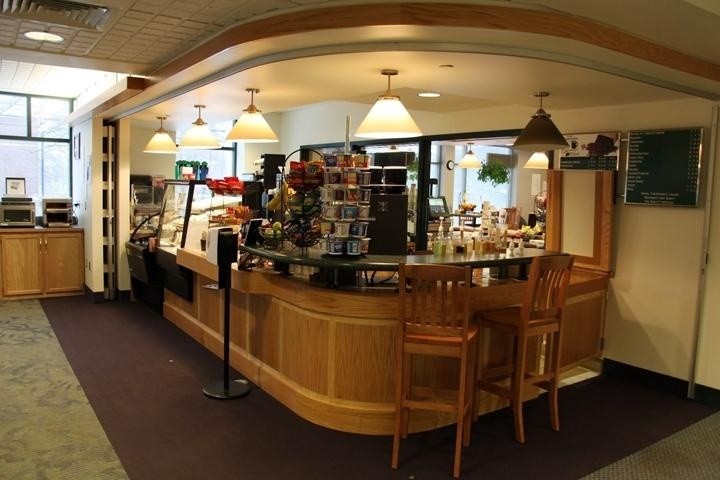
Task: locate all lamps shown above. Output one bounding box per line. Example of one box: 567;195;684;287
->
142;117;178;153
458;142;482;168
524;151;549;170
225;88;280;143
354;69;423;140
512;91;570;151
179;105;223;149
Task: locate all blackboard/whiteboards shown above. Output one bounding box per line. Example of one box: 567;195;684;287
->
624;126;703;207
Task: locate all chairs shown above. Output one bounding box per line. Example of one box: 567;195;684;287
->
473;253;575;444
501;207;522;228
391;262;480;479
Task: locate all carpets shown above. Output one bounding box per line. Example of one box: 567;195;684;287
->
0;298;130;480
581;411;719;479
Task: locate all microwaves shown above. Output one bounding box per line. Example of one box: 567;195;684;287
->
0;201;36;229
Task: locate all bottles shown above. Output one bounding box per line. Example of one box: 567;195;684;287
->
433;216;523;257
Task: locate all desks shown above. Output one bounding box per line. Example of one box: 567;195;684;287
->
450;213;482;239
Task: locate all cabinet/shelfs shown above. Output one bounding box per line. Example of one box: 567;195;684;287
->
0;226;84;301
